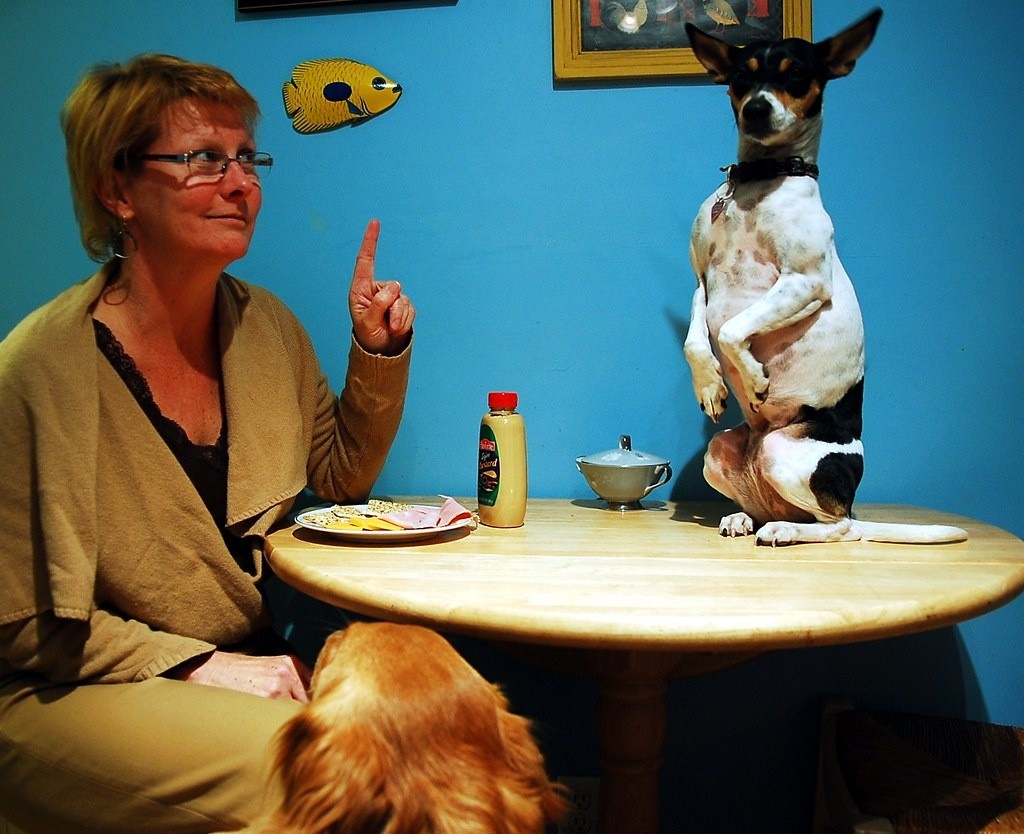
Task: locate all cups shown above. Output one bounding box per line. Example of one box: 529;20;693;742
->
577;435;673;512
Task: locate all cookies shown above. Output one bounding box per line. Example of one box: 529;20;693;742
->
302;500;413;526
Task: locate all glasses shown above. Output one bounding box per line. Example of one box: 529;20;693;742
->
130;149;274;180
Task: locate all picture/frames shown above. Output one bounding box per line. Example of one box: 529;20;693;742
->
551;0;812;81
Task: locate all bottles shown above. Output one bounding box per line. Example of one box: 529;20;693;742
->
479;392;527;528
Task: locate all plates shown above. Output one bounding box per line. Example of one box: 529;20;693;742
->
294;505;473;543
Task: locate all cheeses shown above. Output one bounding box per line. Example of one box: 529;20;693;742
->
325;516;404;531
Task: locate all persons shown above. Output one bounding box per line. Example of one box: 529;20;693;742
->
0;53;415;834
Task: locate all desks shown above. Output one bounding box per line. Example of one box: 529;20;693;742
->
264;501;1024;834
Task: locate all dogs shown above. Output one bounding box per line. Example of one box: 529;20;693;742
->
682;7;969;548
202;618;570;834
